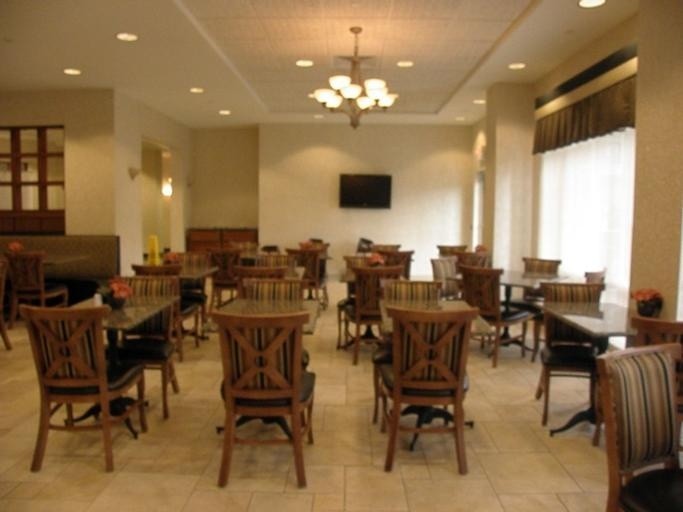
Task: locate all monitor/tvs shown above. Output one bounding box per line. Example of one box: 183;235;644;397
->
339;174;391;209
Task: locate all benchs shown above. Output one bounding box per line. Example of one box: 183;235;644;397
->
1;236;120;306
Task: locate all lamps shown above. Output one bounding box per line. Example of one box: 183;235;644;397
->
313;27;400;129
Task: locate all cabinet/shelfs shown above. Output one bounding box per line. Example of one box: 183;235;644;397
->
190;229;257;253
